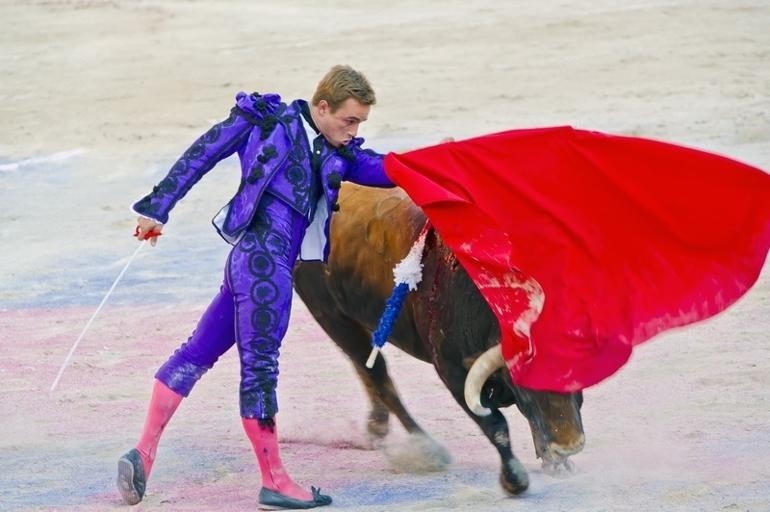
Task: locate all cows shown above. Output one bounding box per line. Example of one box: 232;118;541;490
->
292;182;586;494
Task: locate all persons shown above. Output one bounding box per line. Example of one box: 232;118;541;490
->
117;64;455;510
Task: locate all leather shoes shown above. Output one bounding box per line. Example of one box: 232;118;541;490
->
117;449;146;504
257;485;332;510
